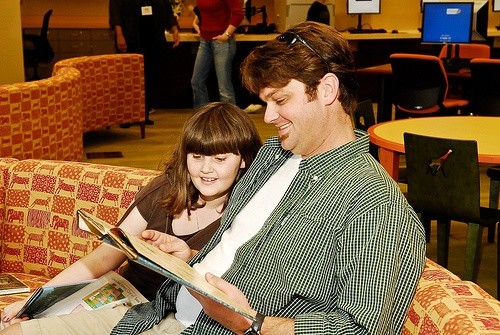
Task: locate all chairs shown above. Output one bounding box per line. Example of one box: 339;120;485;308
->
351;41;500;280
23;10;53;79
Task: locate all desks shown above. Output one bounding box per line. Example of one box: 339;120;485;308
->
355;62;471;124
366;116;500;184
167;29;420;42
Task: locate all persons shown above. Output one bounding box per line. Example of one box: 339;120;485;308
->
0;102;263;332
1;22;427;335
191;0;245;110
109;0;179;129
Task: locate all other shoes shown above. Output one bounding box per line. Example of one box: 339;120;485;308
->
144;119;154;125
242;104;262;113
119;122;132;128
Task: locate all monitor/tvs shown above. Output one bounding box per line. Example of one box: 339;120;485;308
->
347;0;381;29
422;2;475;44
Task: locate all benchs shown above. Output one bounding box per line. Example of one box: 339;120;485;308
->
0;53;146;160
0;158;500;335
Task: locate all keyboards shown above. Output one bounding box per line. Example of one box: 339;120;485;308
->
349;29;387;33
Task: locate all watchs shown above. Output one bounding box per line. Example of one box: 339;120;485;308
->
244;313;266;335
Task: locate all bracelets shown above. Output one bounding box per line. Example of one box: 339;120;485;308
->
225;32;232;38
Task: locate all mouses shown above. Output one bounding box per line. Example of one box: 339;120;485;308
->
392;30;398;33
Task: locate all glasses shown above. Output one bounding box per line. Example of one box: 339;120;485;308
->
275;31;330;74
